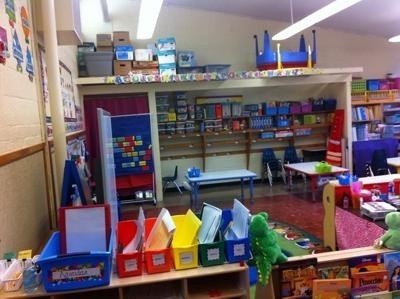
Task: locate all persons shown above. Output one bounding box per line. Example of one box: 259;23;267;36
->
390;265;400;291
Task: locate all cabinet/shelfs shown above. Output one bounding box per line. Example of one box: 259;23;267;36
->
159;110;334;175
351;98;400;141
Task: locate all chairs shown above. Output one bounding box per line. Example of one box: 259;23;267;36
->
162;165;183;196
365;149;391;176
262;147;307;186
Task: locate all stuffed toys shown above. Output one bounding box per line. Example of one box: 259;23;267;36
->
373;211;400;251
248;211;288;288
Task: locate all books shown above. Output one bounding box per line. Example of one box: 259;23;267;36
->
362;200;398;212
352;105;383;142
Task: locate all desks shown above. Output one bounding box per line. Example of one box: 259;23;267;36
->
283;162;350;201
184;168;257;212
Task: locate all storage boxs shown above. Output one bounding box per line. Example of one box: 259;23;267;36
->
77;31;232;77
